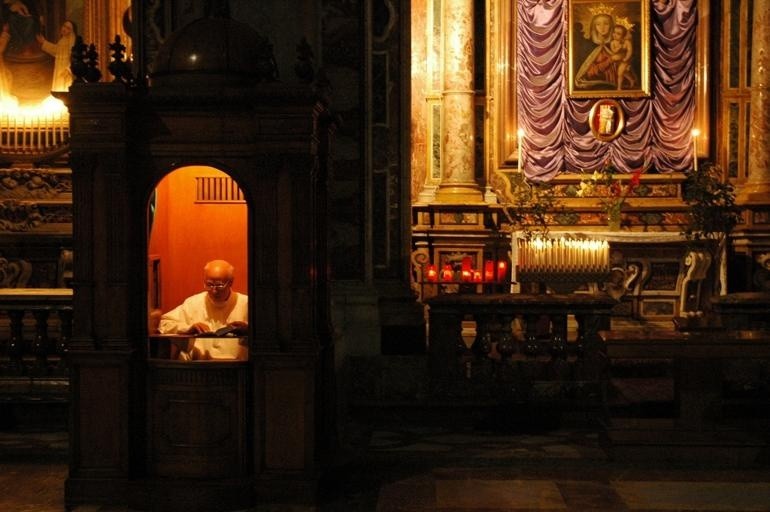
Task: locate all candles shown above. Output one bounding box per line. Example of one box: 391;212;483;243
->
516;236;609;273
425;256;508;284
1;116;63;155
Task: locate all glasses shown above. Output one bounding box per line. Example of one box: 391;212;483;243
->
203;281;231;290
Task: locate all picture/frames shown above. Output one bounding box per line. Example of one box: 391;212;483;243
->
567;0;654;100
0;0;108;134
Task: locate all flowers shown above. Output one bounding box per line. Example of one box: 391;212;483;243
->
575;166;644;222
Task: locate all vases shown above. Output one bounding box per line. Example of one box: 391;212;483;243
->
606;216;620;232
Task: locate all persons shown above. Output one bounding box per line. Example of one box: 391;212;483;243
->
574;14;636;91
157;260;248;361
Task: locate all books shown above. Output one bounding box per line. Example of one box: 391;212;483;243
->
194;325;237;337
